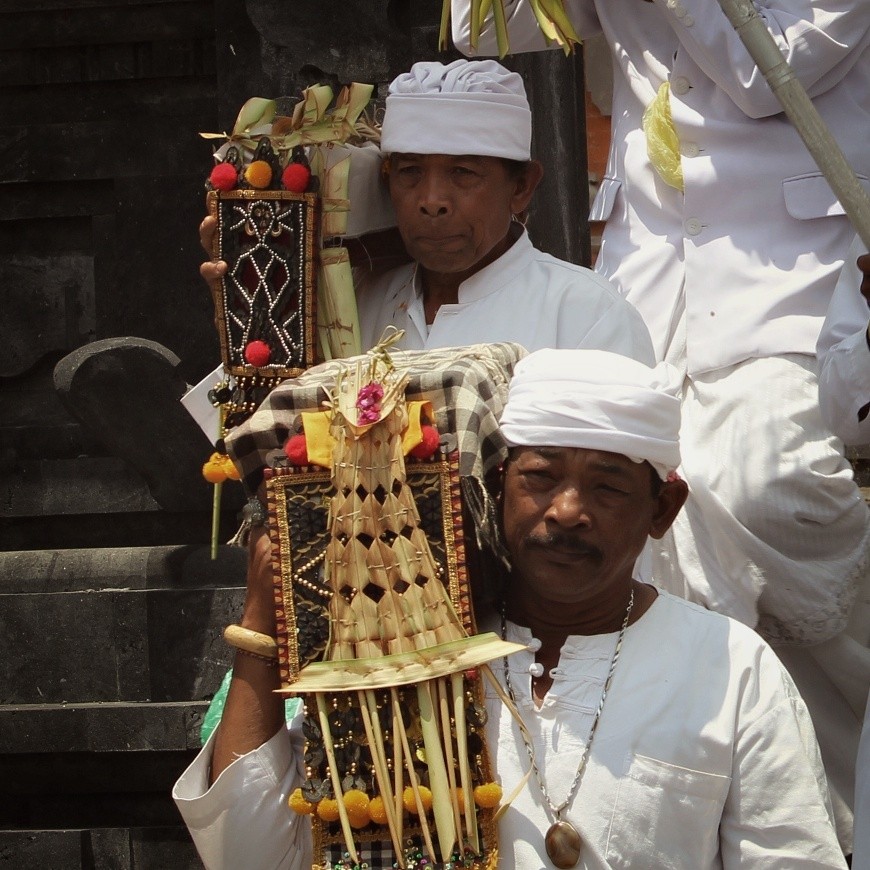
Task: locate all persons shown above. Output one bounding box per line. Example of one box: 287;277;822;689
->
448;2;870;870
172;356;850;869
200;60;657;370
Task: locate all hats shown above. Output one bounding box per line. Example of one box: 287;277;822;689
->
381;61;534;162
501;350;682;481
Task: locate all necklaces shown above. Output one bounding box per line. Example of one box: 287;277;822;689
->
493;579;640;869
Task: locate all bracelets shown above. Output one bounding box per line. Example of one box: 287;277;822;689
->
223;624;279;661
